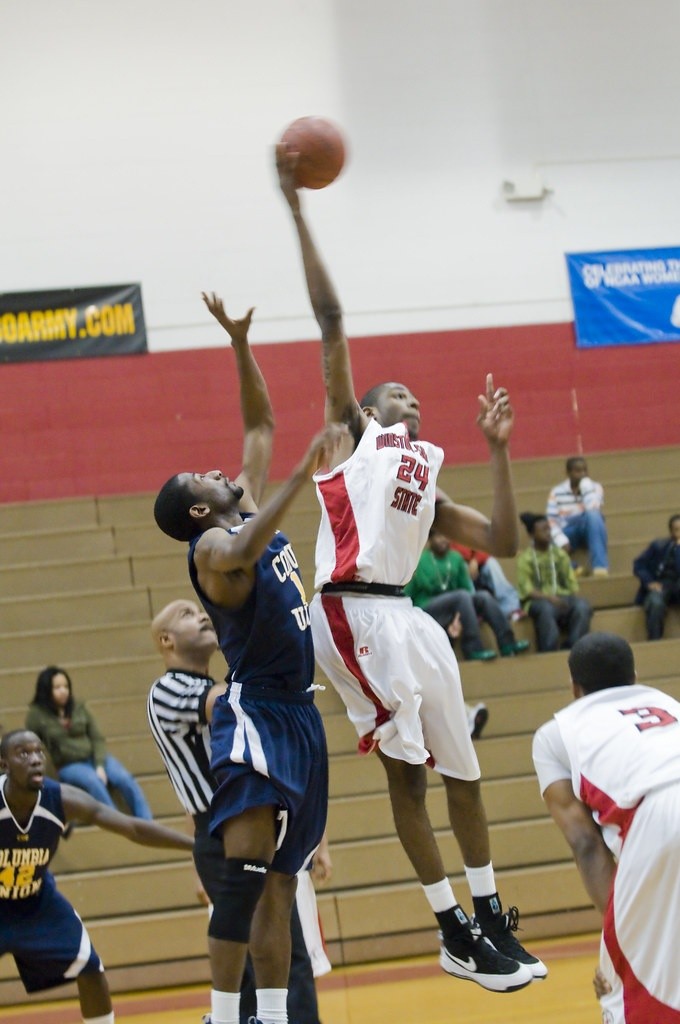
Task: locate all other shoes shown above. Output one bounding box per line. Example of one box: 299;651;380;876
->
470;702;489;742
469;650;497;661
574;566;585;578
501;640;530;658
593;566;608;578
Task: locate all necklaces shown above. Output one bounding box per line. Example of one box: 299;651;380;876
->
431;549;451;589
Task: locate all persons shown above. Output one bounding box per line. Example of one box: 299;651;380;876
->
531;630;680;1024
632;514;680;642
0;729;194;1024
153;291;341;1024
450;543;521;623
405;530;530;661
148;599;320;1024
24;666;153;821
275;143;549;992
516;510;593;653
547;456;610;576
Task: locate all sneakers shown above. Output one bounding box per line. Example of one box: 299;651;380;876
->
471;906;549;978
437;923;533;993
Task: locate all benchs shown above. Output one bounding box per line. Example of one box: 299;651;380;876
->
0;448;680;1006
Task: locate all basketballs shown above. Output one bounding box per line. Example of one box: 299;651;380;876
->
276;115;351;192
